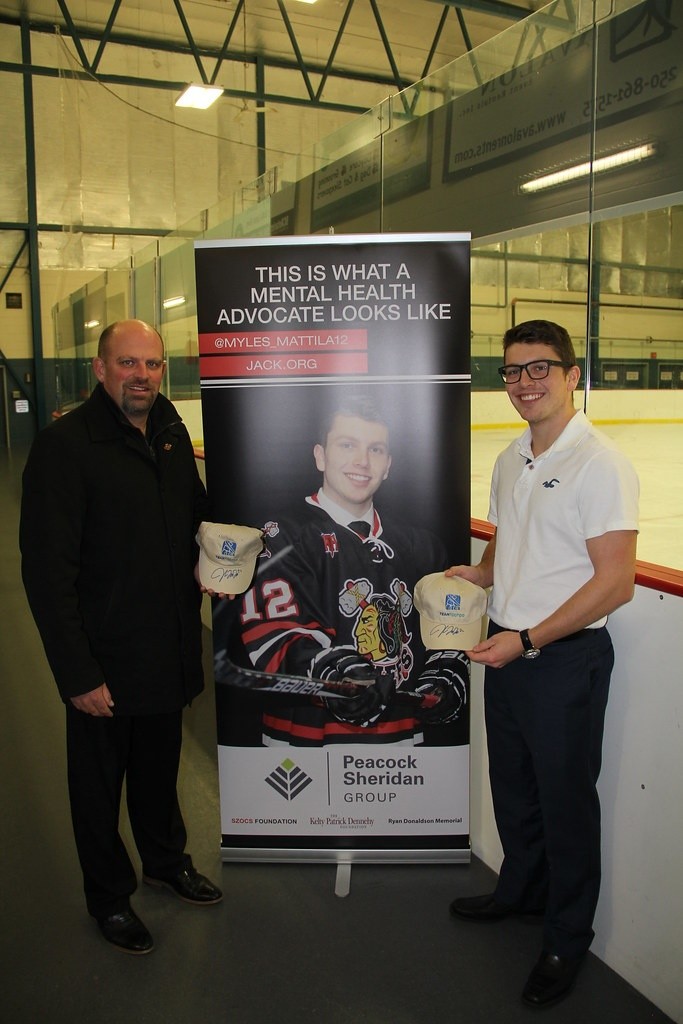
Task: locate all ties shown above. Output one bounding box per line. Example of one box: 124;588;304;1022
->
347;521;372;539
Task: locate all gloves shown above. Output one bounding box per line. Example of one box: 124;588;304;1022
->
414;648;471;725
305;643;397;728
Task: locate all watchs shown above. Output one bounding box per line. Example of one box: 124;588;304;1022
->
520;629;541;661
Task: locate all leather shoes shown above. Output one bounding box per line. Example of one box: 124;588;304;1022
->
521;942;591;1008
448;892;545;923
90;895;153;954
142;866;223;904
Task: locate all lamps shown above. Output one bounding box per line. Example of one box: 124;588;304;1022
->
176;83;225;110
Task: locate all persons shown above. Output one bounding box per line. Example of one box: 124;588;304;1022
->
20;319;237;954
446;321;640;1009
232;395;470;747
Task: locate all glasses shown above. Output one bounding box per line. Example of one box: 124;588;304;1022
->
497;359;573;383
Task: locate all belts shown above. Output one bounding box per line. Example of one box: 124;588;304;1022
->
490;618;606;642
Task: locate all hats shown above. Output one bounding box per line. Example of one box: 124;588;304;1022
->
195;522;263;594
413;572;488;652
